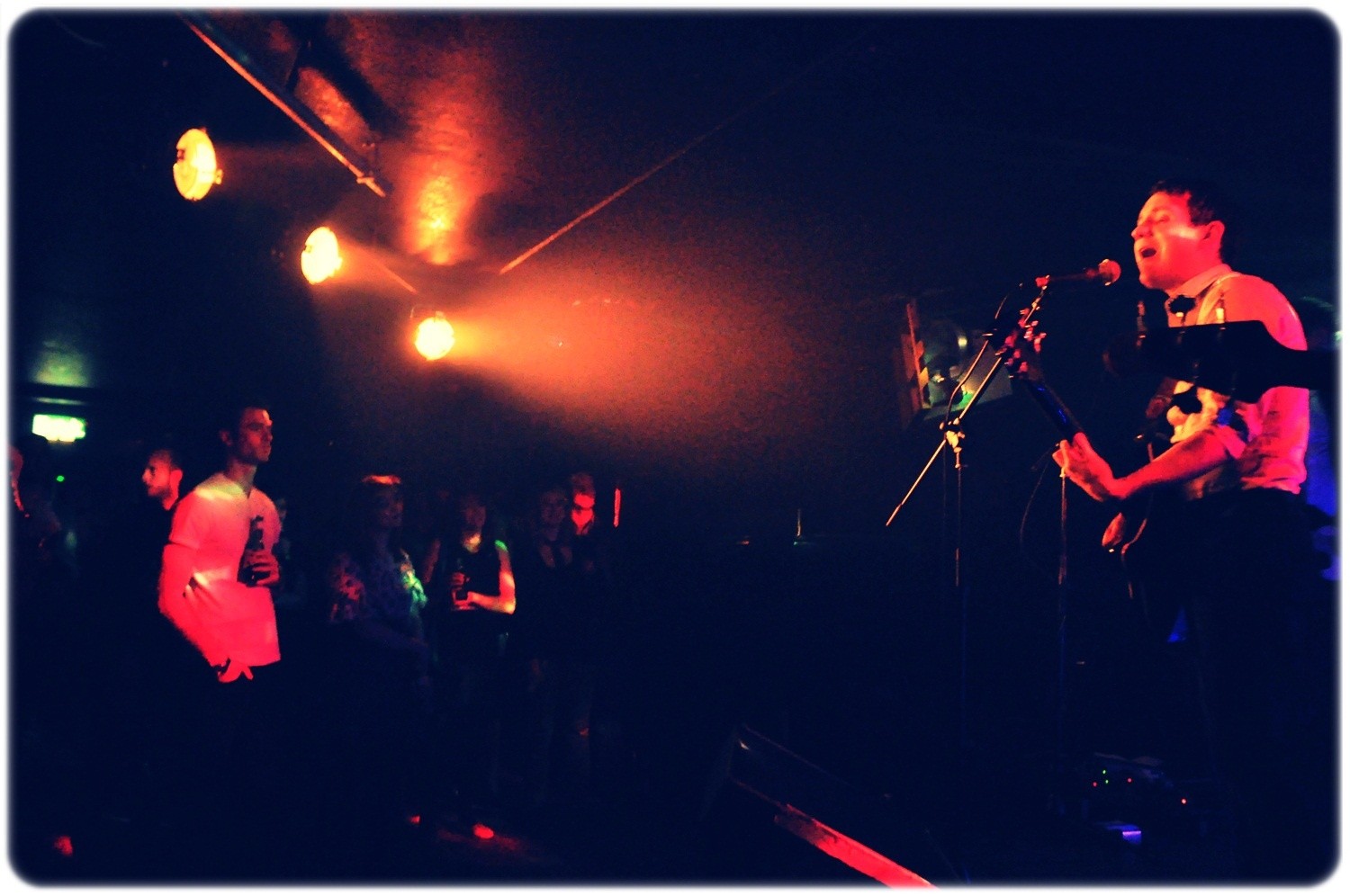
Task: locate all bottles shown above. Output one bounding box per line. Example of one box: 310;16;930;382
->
239;516;264;586
455;558;468;599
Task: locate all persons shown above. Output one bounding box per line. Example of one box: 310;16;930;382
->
10;396;623;852
1050;172;1311;885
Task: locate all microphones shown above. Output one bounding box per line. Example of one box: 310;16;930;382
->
1035;258;1122;287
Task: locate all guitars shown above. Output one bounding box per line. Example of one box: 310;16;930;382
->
989;295;1184;596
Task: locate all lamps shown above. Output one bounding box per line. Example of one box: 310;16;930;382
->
124;109;223;210
400;301;454;363
274;214;345;284
